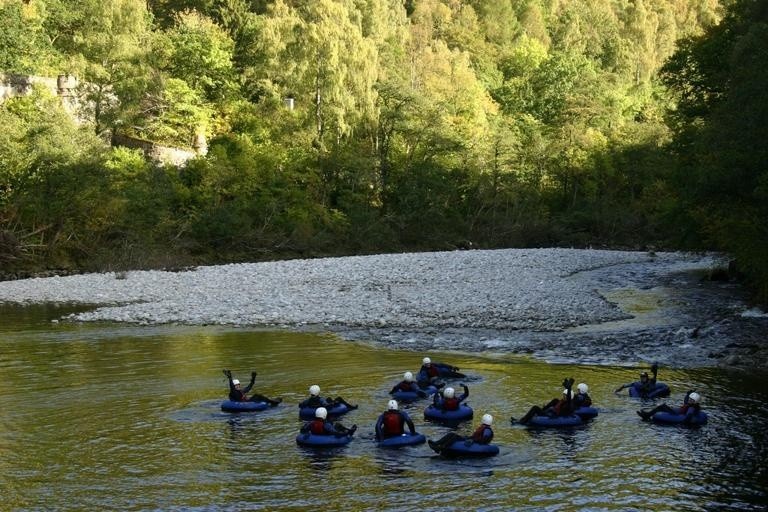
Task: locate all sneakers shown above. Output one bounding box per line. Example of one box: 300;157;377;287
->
636;410;650;421
349;424;357;435
272;397;282;407
510;416;520;425
427;439;441;454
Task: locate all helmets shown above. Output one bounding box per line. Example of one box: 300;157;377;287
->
388;400;399;410
423;357;431;364
315;407;327;420
233;379;240;386
481;413;493;426
444;387;455;399
404;372;413;382
577;382;589;394
564;388;576;400
309;385;321;397
639;372;648;378
689;392;700;401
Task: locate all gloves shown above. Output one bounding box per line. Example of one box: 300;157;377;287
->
454;366;460;371
251;372;256;378
418;390;427;399
683;415;692;424
687;388;697;394
464;439;474;446
222;369;232;376
459;383;466;387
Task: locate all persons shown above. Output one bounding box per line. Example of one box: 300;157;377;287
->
616;365;658;396
376;399;415;438
223;368;282;407
428;413;494;454
388;371;427;400
510;378;575;426
299;385;358;411
303;406;357;437
574;383;592;408
433;383;469;411
636;390;701;422
419;357;454;388
